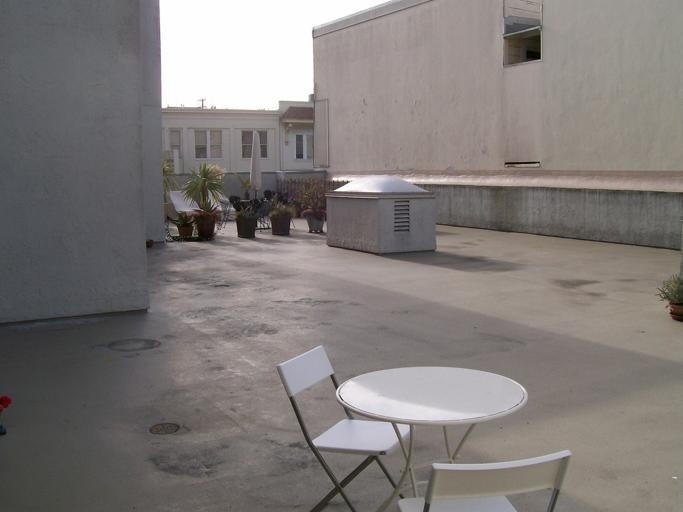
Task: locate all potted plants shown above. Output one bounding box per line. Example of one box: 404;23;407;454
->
164;166;327;237
654;272;683;322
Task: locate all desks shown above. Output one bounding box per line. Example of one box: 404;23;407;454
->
335;365;527;512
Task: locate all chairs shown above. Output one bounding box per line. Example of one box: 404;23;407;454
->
275;344;413;512
396;449;573;512
